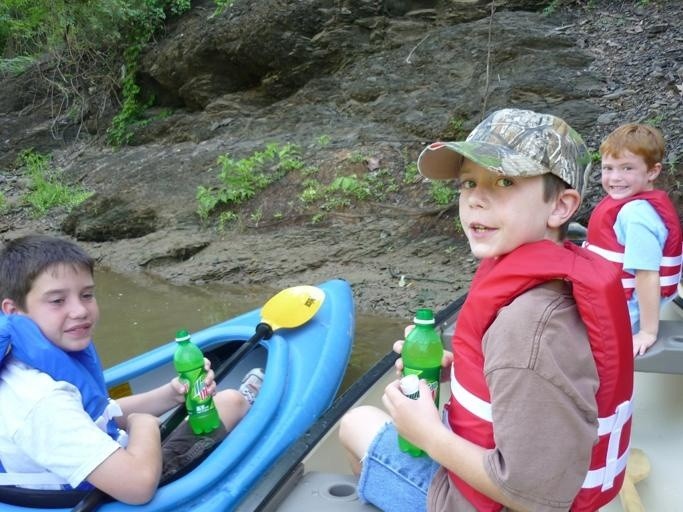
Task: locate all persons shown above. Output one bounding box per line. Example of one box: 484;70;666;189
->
337;105;637;512
578;122;683;358
0;231;267;512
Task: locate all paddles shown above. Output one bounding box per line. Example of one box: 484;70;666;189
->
74;285;325;508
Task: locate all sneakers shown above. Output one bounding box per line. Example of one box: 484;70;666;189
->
240;366;264;402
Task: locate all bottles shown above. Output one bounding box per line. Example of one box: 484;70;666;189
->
172;329;221;435
399;308;447;458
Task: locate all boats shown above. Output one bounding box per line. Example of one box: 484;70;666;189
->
231;218;682;512
1;276;356;512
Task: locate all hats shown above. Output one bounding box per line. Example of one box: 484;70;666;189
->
418;107;593;203
